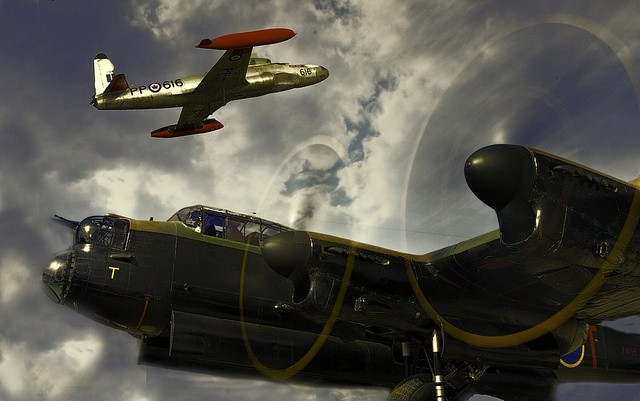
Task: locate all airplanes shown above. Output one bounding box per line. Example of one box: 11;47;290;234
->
42;144;640;401
91;27;329;138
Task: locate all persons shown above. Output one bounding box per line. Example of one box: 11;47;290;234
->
199;215;216;237
